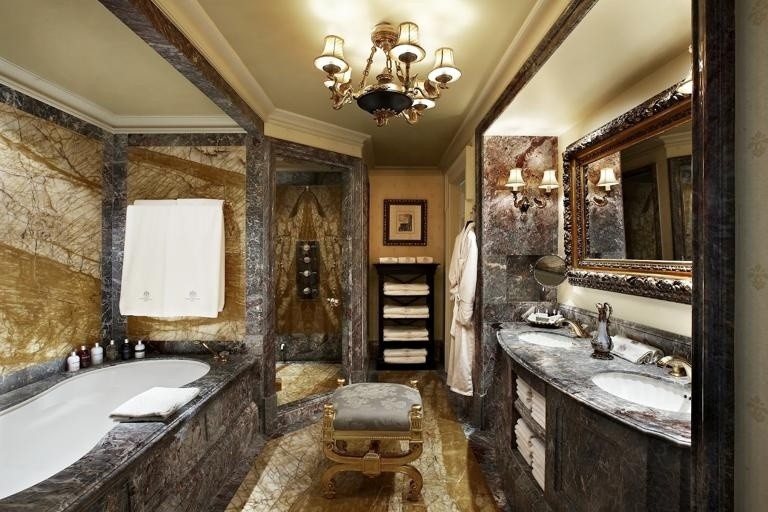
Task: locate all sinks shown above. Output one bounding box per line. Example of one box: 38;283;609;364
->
593;368;692;413
518;330;573;347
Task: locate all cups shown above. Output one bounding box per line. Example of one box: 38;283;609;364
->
219;351;229;362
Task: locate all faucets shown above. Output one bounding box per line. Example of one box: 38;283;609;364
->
193;339;220;359
556;315;590;338
657;354;692;380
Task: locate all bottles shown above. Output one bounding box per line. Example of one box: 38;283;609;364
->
590;300;615;359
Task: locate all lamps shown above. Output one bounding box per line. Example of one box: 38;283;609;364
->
587;166;620;207
504;167;559;213
313;21;464;130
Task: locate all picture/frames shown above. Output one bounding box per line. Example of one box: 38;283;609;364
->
382;198;429;246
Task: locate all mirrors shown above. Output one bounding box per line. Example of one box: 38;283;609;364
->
560;72;693;305
528;253;568;287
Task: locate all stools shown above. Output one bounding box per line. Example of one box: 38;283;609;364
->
319;375;426;500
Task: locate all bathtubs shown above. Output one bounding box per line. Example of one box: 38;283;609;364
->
0;356;211;503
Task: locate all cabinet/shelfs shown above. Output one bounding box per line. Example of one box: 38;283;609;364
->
371;261;441;370
508;370;545;494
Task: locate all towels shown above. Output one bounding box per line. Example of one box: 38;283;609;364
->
382;281;429;365
108;386;201;421
513;376;546;491
380;256;434;264
116;197;226;320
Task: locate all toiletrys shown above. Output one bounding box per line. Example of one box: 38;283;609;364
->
67;339;145;372
